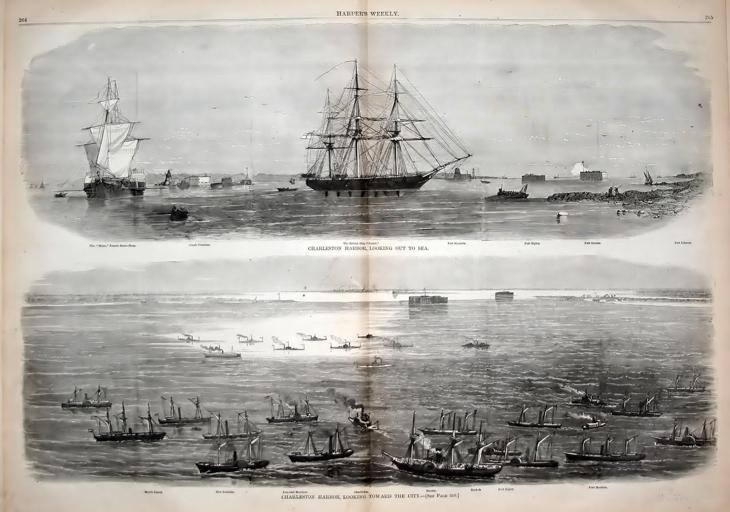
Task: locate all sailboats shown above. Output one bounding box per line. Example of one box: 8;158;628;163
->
566;372;716;462
61;384;210;441
74;73;146;198
382;405;563;478
303;56;471;196
287;422;354;462
195;407;270;477
265;393;318;424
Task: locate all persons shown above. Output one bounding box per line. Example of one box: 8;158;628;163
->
614;187;619;197
608;186;613;197
349;412;367;428
424;448;448;463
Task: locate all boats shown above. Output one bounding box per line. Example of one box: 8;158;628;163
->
486;184;529;201
463;338;490;349
178;332;414;368
346;402;379;432
277;187;297;192
170;204;187;221
